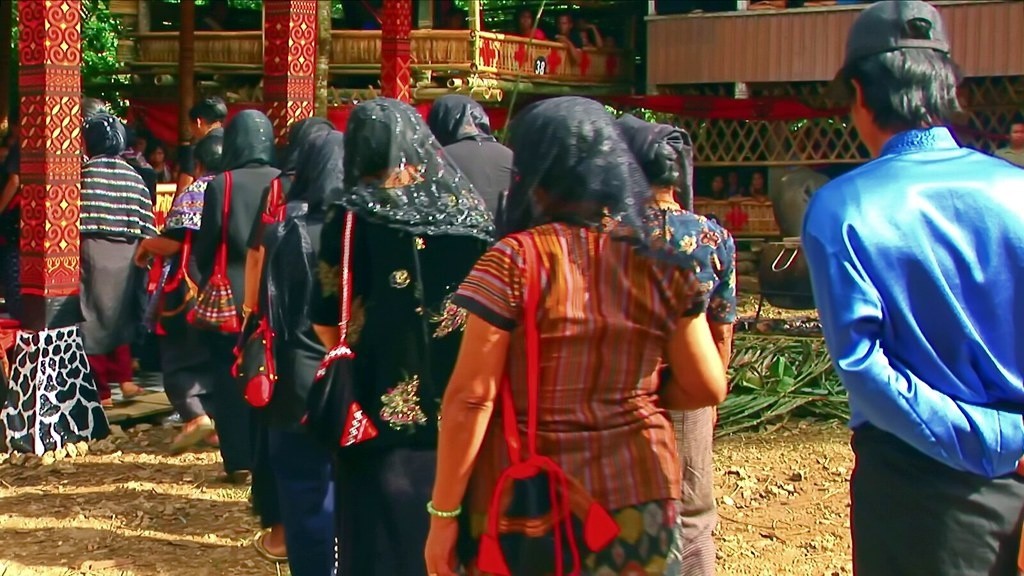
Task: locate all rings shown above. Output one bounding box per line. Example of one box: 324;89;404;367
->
429;573;437;576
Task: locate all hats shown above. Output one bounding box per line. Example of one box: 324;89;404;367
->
828;0;953;104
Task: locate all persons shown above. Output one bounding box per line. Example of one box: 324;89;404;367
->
710;169;768;203
511;8;615;63
993;121;1024;168
0;93;738;576
801;0;1024;576
747;0;788;11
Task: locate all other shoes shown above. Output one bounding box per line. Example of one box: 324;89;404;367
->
166;413;216;455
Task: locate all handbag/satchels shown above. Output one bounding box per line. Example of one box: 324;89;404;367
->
230;318;278;408
299;345;379;450
476;458;620;576
155;264;241;338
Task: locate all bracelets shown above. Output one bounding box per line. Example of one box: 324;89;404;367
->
426;501;462;518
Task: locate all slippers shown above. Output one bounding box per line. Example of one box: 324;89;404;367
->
100;403;114;410
252;527;291;563
124;383;144;400
